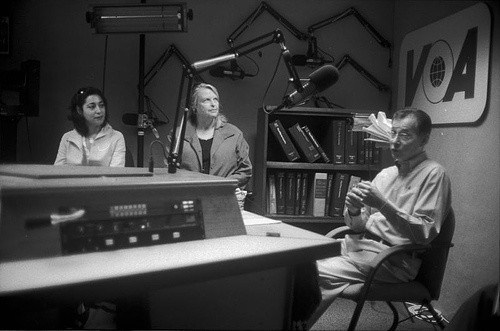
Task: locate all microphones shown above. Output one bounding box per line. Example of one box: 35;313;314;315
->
122;113;168;125
209;66;256;79
267;64;338;114
292;54;333;66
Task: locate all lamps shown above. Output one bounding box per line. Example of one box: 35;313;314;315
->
86;3;193;34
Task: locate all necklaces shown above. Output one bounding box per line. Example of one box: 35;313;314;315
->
197;130;210;144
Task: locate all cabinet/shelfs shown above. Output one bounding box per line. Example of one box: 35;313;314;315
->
252;105;384;235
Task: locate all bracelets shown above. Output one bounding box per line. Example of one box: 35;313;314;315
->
379;200;388;211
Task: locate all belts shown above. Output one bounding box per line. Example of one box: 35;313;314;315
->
364;230;393;248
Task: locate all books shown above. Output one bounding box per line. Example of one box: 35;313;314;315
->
267;117;381;217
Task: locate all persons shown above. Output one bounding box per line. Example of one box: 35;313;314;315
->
54;86;126;166
163;83;252;188
297;107;451;331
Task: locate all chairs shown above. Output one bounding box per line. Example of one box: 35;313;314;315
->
317;205;455;331
